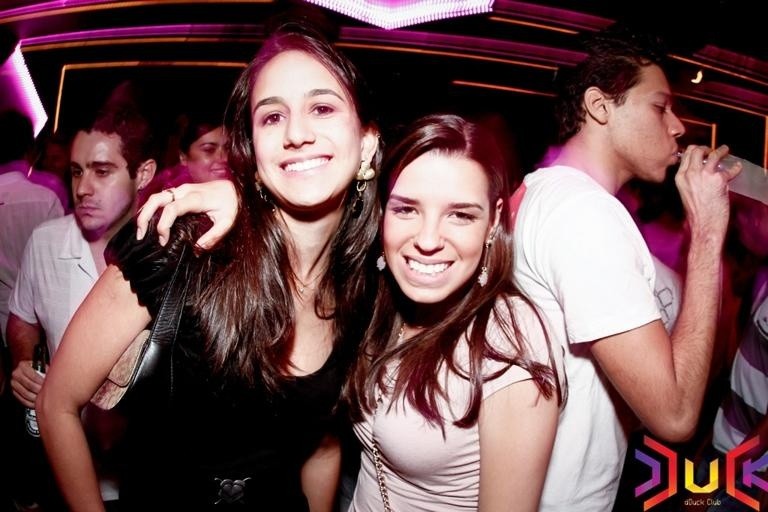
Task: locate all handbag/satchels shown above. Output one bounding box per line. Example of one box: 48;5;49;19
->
81;215;202;442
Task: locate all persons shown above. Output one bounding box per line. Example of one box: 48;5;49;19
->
1;24;768;512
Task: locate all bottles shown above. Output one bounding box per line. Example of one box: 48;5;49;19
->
677;148;768;205
24;343;50;439
